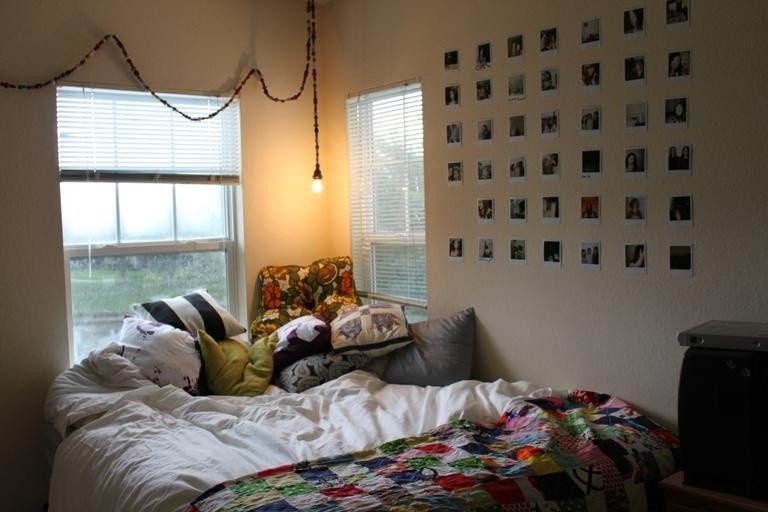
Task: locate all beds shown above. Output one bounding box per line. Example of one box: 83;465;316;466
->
46;378;680;511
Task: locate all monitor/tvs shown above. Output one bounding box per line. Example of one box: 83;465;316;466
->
677;348;768;502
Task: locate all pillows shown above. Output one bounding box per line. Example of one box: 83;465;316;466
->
196;328;278;395
371;350;391;378
329;305;415;359
305;255;354;306
117;315;203;395
132;288;247;341
383;307;475;385
271;313;329;373
251;303;315;342
313;292;364;323
259;263;312;306
275;350;370;395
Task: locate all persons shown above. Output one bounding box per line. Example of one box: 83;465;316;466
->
446;2;691;269
